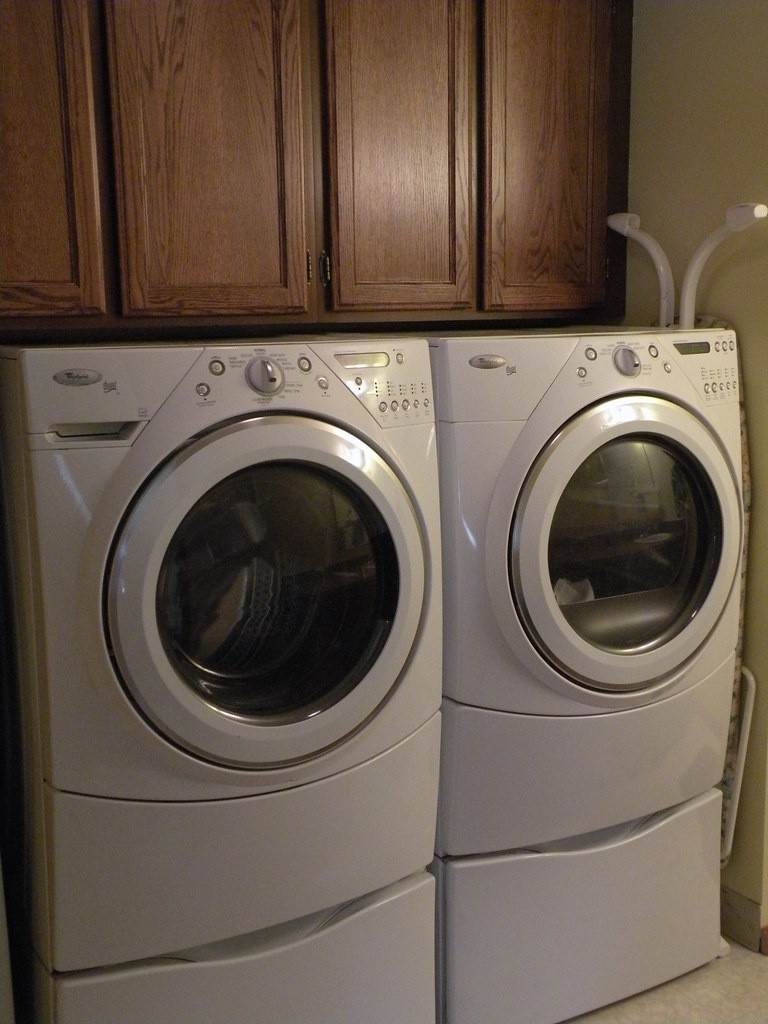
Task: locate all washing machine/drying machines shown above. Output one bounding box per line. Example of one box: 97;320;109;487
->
1;335;444;1024
421;319;751;1022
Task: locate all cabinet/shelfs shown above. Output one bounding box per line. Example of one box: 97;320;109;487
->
2;1;628;325
